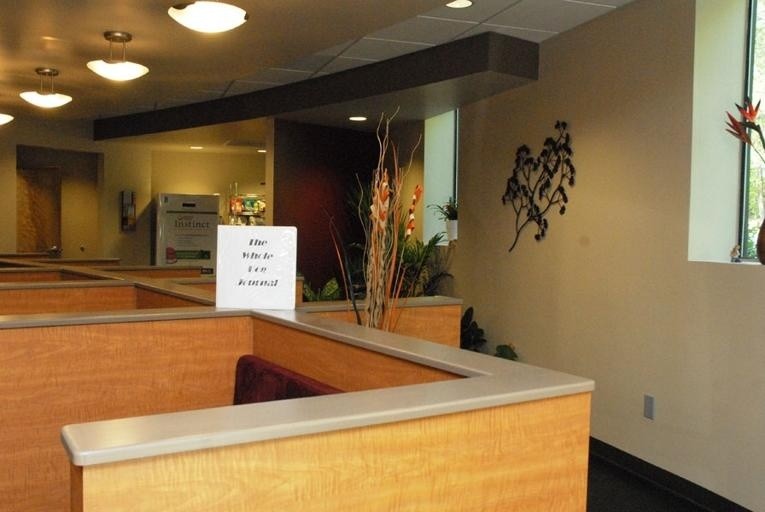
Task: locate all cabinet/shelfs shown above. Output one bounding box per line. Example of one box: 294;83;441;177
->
229;194;268;226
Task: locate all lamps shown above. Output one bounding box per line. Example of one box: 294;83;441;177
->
19;67;73;109
86;31;149;81
167;0;251;34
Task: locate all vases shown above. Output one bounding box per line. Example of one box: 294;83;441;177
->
756;218;764;264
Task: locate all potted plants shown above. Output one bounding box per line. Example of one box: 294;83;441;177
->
426;197;459;245
